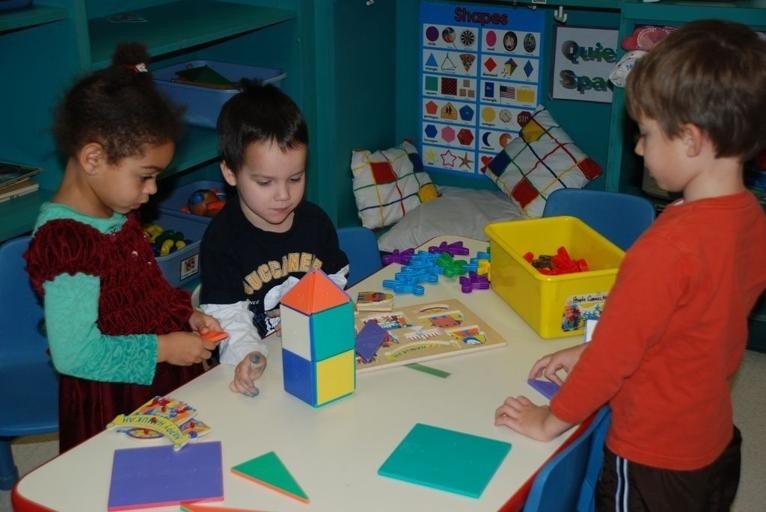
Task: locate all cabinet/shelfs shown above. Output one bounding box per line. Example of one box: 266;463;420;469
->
2;0;317;246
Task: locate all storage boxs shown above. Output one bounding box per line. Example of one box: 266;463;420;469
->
485;215;627;340
139;181;228;287
147;60;288;132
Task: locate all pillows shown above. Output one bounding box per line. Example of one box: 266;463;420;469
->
349;100;602;253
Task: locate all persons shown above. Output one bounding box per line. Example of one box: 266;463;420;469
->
196;81;352;398
495;19;766;511
20;63;223;457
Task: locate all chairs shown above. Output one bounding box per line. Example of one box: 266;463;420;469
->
0;237;63;493
522;407;612;512
543;188;656;253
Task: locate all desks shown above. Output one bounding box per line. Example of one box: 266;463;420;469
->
13;233;589;511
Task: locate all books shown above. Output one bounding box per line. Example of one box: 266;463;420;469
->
0;161;43;204
